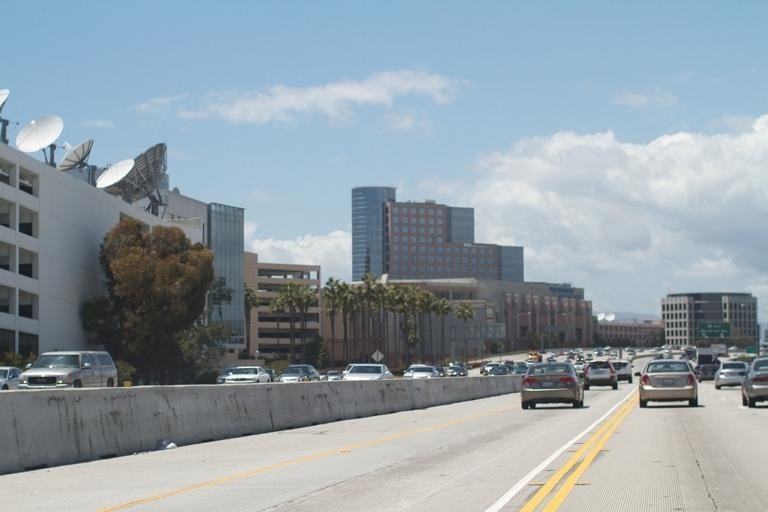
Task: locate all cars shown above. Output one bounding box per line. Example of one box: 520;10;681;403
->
698;364;720;382
573;364;585;377
527;345;695;362
634;359;698;407
0;365;25;390
610;360;634;383
741;357;768;408
520;361;585;408
692;366;700;377
714;360;749;389
731;352;756;362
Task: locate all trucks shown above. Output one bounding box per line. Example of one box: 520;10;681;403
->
696;346;719;365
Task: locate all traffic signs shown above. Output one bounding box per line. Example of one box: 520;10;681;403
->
696;321;730;338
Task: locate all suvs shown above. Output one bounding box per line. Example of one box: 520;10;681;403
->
582;359;619;391
16;350;119;391
